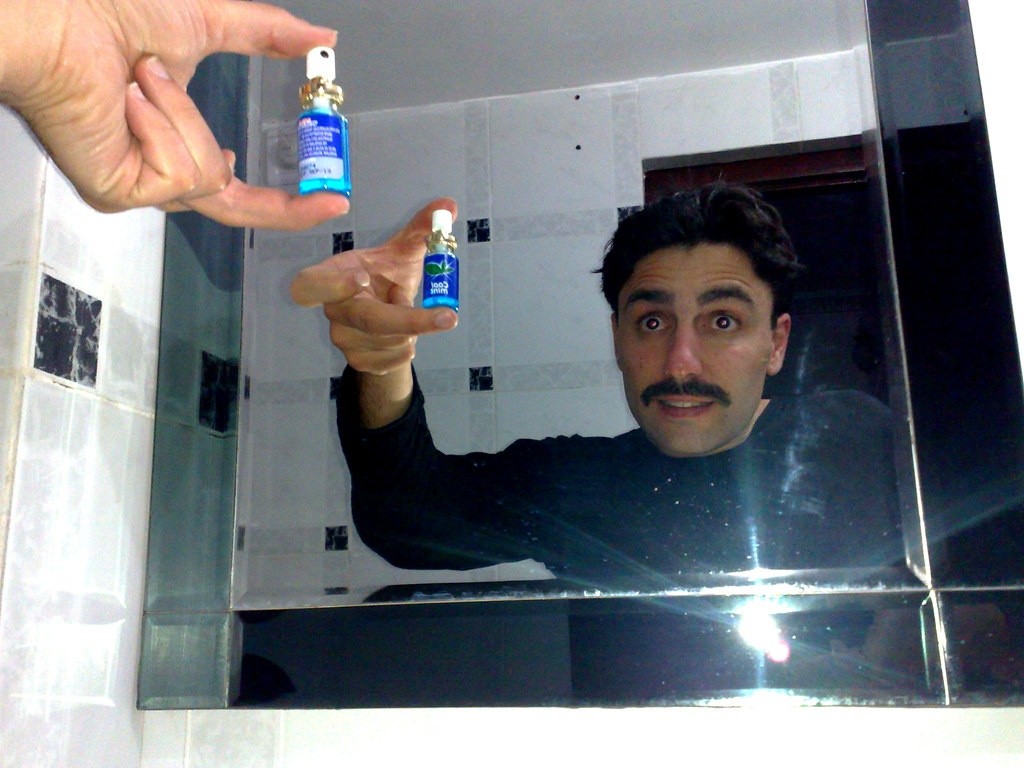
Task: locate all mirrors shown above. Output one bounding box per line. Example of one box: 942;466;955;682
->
136;0;1024;709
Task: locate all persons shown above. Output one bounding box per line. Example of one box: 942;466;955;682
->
0;0;350;232
291;178;1024;701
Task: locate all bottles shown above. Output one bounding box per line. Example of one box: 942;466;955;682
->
421;208;460;312
296;46;353;200
